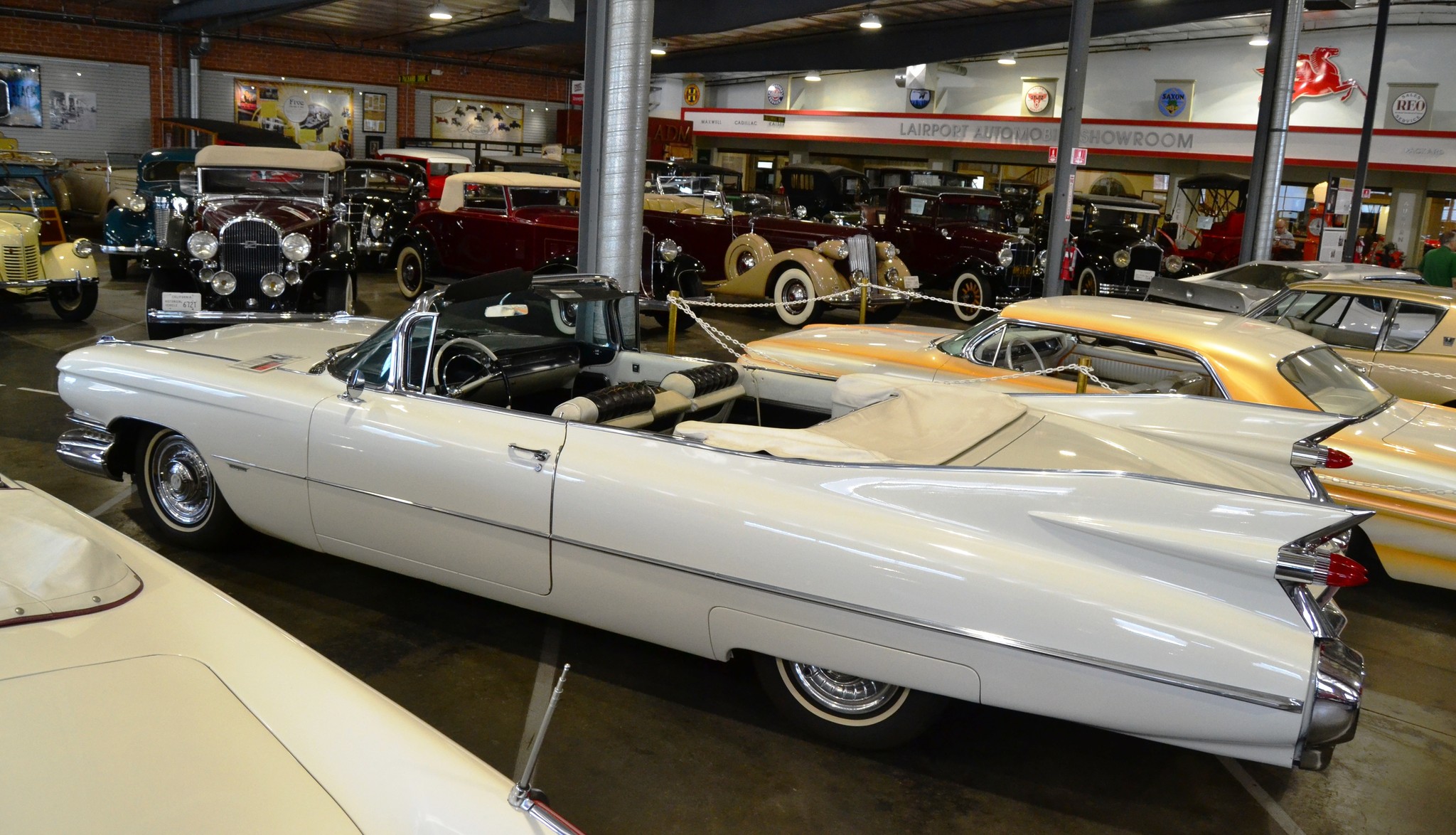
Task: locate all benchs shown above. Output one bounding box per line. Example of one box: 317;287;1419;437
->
641;197;747;217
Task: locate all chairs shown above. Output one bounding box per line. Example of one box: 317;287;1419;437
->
550;361;746;435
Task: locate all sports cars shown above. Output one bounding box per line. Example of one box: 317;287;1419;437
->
53;273;1375;776
732;293;1456;603
1167;260;1432;315
1236;279;1456;410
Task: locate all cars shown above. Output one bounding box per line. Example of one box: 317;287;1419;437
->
0;111;1175;341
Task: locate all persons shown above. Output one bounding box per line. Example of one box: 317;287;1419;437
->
1272;219;1296;249
1417;230;1456;288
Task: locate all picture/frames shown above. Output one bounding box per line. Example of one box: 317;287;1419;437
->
362;91;387;134
0;61;43;129
365;134;384;159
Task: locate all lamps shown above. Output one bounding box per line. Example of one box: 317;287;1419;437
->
429;0;453;21
859;4;881;29
1248;23;1268;46
998;50;1016;66
804;70;822;82
650;38;668;56
